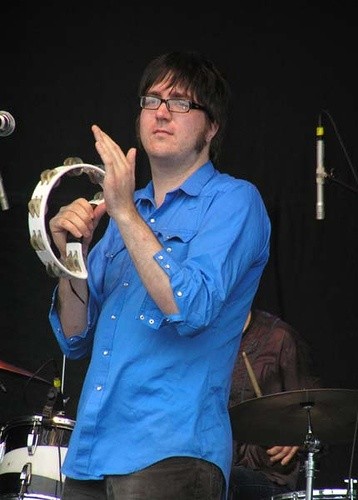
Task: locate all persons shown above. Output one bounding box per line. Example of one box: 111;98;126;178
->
227;306;308;499
48;49;272;498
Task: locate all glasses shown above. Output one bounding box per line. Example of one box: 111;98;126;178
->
140;94;214;123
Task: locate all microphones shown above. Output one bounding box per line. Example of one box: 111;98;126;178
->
0;110;16;137
54;374;66;417
316;113;327;220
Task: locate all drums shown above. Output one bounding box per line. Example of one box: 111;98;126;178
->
271;489;356;500
0;415;77;500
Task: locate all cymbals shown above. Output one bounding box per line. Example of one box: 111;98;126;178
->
28;158;106;279
229;388;358;445
